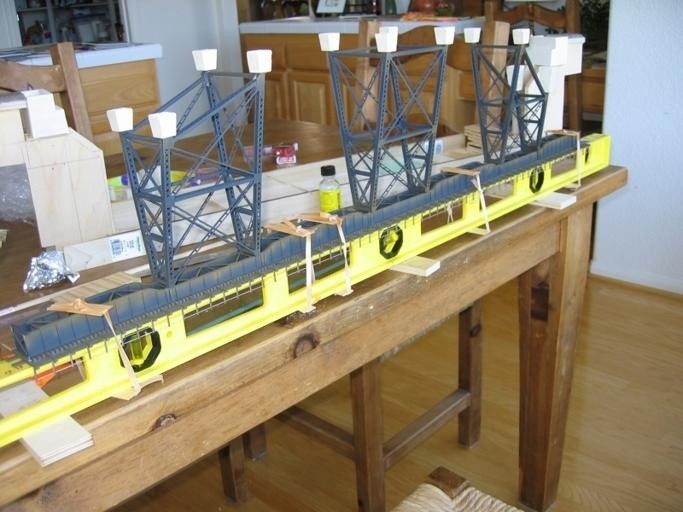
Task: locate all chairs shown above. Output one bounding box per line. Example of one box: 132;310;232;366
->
241;19;511;510
3;43;250;509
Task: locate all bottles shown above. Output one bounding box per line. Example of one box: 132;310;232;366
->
317;164;342;214
243;142;299;161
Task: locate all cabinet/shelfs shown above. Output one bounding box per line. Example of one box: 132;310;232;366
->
238;12;589;133
1;39;163;156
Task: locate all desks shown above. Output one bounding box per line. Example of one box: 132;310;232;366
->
0;117;628;509
